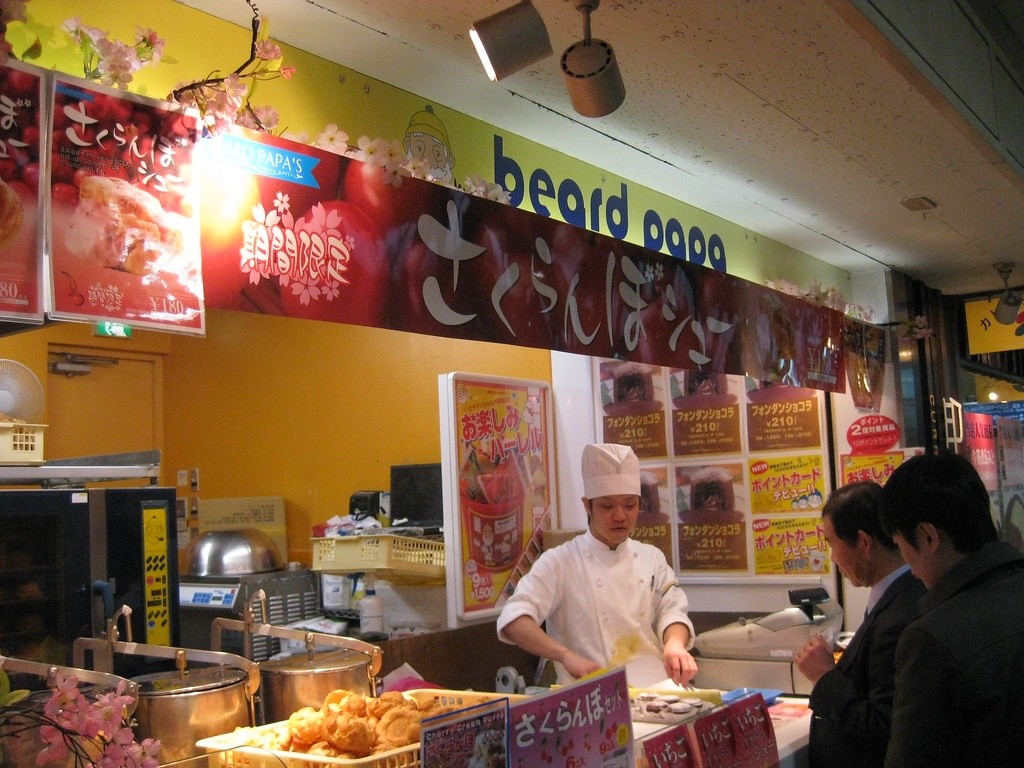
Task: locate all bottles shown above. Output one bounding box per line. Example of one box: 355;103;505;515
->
359;588;383;632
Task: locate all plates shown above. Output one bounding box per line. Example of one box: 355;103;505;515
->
724;687;784;708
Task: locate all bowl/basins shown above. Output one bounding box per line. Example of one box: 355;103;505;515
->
180;528;287;577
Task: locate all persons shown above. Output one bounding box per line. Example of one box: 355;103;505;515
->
793;480;931;768
875;451;1024;768
497;441;700;689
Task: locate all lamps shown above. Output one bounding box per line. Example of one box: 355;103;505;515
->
993;261;1023;325
560;0;626;118
468;0;554;82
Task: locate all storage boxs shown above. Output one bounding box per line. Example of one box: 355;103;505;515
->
0;422;50;466
310;533;446;586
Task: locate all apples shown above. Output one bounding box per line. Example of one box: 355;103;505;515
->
158;127;741;373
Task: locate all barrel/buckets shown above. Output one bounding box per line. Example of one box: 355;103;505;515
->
264;650;372;722
129;667;252;760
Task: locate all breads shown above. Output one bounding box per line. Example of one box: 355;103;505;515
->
0;178;25;251
238;689;495;768
67;177;179;273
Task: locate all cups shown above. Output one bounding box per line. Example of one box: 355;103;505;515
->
461;488;524;571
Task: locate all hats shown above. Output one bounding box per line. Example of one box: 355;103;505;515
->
581;443;641;500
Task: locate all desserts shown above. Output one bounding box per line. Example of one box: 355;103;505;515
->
683;368;727;397
640;471;660;512
689;468;734;511
613;361;655;400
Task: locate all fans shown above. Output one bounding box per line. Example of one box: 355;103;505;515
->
0;359;45;424
